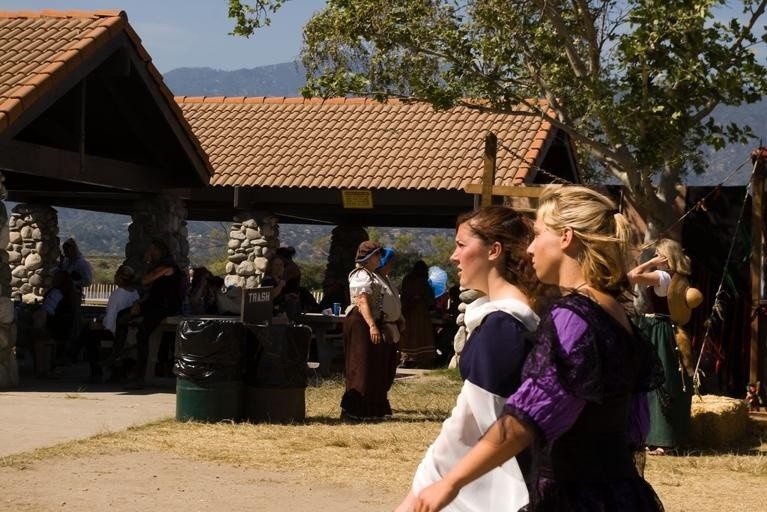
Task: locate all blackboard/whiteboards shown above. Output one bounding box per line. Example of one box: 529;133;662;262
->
240;285;274;323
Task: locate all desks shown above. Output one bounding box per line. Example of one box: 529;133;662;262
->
299;311;348;377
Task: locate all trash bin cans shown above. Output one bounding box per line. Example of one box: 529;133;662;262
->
175;319;312;425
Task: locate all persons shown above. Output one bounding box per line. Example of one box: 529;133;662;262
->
392;205;558;511
397;260;462;369
607;203;653;449
339;240;393;425
372;246;407;417
624;236;694;457
404;179;665;511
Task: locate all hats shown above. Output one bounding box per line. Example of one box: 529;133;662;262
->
666;270;705;327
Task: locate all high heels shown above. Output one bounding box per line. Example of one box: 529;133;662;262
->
648;446;680;456
